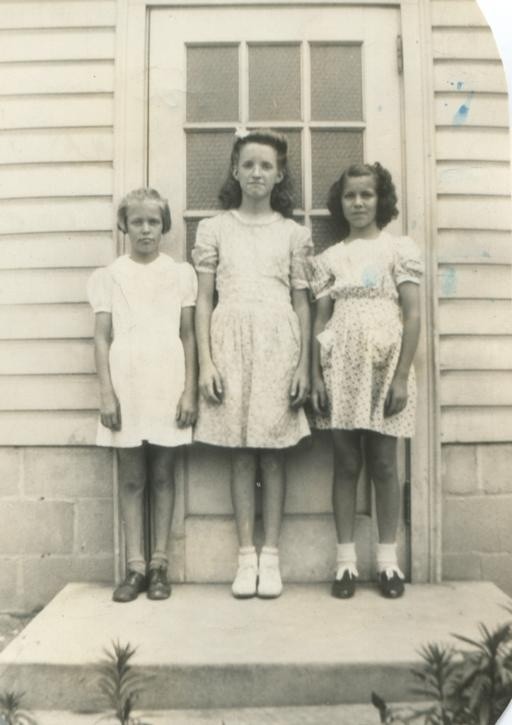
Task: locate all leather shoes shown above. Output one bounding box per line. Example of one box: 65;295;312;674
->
113;572;147;602
377;569;404;598
332;569;356;598
148;567;172;600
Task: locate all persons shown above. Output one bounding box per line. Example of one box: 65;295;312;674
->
186;126;314;598
85;186;198;604
304;159;427;600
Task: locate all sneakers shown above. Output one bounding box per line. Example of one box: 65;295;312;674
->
231;561;257;598
257;562;283;598
